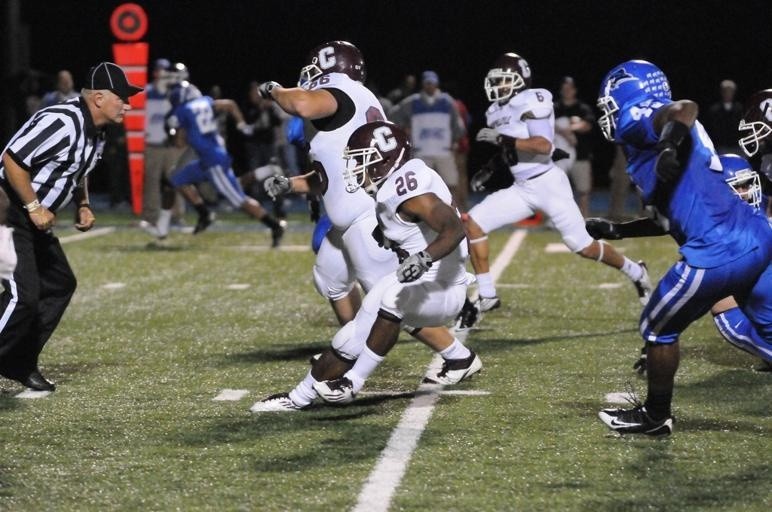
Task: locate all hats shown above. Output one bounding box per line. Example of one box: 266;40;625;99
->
81;62;143;99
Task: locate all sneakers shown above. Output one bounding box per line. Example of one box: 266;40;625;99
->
193;211;216;233
140;221;164;241
0;366;55;392
634;260;653;305
453;305;482;332
249;392;302;412
472;296;502;313
597;382;674;435
422;349;482;386
272;220;286;248
314;374;355;404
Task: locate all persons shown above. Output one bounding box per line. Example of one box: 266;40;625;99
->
257;40;485;385
584;57;772;437
250;121;471;415
0;60;144;392
17;59;309;249
710;90;771;363
462;52;653;310
378;72;772;223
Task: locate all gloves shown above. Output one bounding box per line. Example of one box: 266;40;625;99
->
263;175;292;202
585;218;619;240
237;122;255;135
476;127;516;149
470;161;497;193
397;250;433;283
654;144;681;186
257;81;281;100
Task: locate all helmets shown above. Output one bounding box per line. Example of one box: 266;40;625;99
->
484;52;533;103
718;153;762;209
153;59;190;99
342;120;413;193
299;40;368;88
738;88;772;164
597;59;672;143
